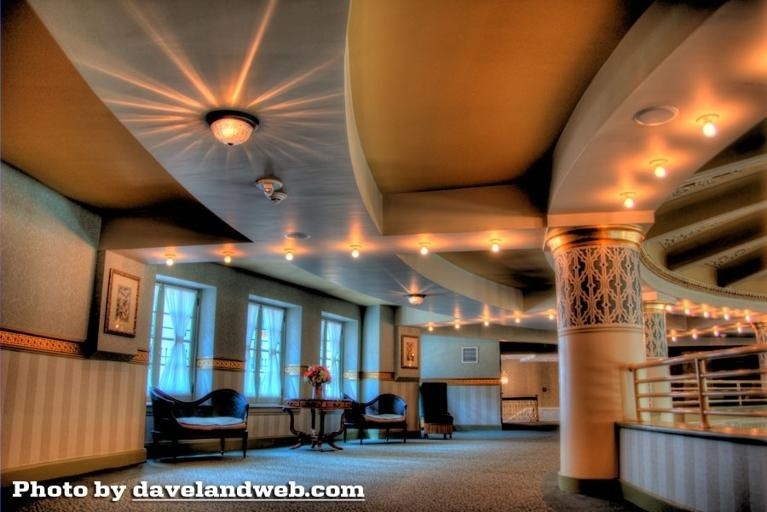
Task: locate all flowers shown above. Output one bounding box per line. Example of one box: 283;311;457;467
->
303;364;331;386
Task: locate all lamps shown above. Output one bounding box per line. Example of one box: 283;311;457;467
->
164;237;502;266
204;109;260;147
255;178;283;197
268;192;287;205
619;114;718;208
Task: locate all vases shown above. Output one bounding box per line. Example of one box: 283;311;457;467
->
312;385;323;399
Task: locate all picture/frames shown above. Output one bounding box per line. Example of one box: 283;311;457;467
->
401;335;420;368
104;268;141;338
460;346;479;364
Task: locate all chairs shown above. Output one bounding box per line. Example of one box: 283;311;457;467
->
420;382;453;439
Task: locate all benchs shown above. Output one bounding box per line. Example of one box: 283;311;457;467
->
342;393;408;445
150;387;250;463
